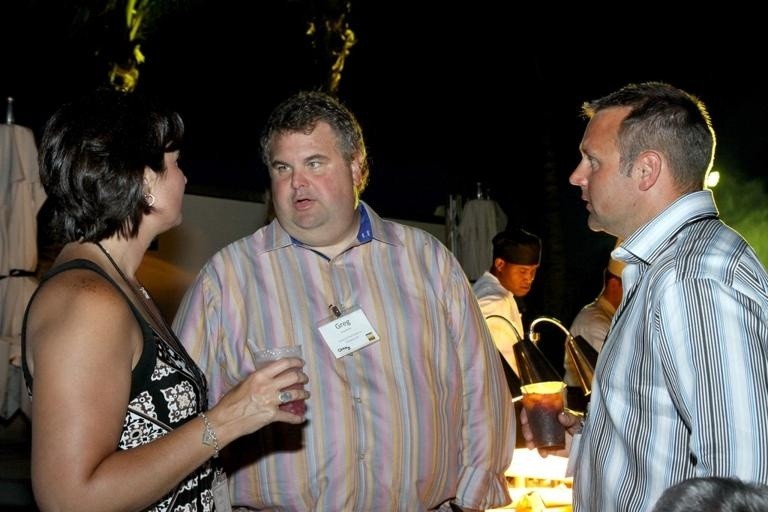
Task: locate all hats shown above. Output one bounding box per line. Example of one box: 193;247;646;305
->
492;226;542;266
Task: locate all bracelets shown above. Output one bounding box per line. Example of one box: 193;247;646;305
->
194;409;221;463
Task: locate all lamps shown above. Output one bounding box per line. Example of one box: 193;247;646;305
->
484;315;600;398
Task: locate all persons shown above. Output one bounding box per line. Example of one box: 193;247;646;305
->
651;474;768;511
562;268;625;414
13;85;308;510
164;85;521;510
469;220;544;390
517;80;768;510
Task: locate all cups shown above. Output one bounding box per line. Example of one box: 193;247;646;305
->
519;380;567;451
252;345;308;418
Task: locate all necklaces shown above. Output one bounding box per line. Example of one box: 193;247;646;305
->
100;246;154;302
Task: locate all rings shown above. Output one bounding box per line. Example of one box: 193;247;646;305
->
277;390;294;408
